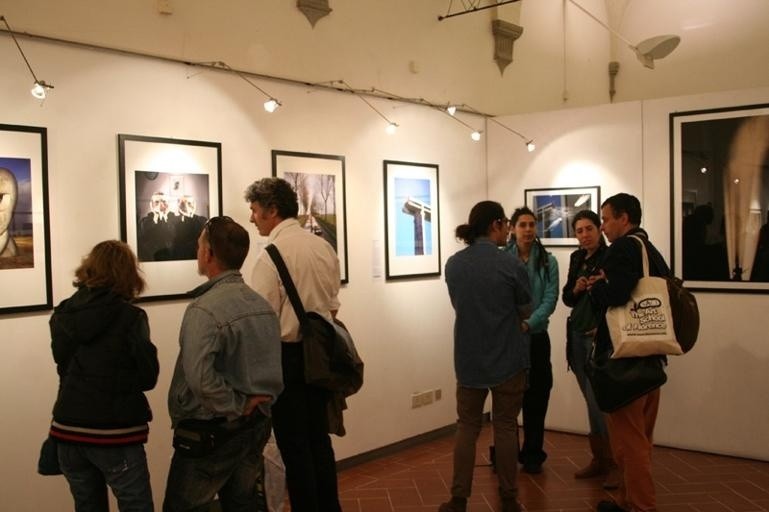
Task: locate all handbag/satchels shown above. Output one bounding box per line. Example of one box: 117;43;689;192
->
606;235;681;356
586;347;668;411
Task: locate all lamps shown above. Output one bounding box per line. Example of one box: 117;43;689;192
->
525;138;538;155
28;78;55;107
260;97;283;113
630;34;680;69
386;122;404;138
468;128;482;142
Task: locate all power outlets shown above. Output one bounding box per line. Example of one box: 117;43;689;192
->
421;389;434;405
410;392;422;409
432;387;443;403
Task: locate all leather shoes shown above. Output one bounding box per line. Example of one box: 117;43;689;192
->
577;465;603;477
502;499;521;512
439;498;465;512
525;459;545;472
606;469;624;488
598;500;621;511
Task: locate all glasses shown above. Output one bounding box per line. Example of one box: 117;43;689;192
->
206;215;235;255
494;219;511;228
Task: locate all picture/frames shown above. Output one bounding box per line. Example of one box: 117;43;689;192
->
666;102;768;294
0;121;55;315
114;132;227;303
382;159;442;281
523;186;603;250
271;149;350;284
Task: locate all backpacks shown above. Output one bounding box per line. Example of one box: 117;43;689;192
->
633;236;699;355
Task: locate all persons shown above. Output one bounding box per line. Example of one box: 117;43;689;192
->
440;201;530;512
244;177;344;512
491;208;559;473
144;191;175;257
176;195;207;256
163;217;282;512
49;240;161;512
562;209;617;490
587;194;677;512
